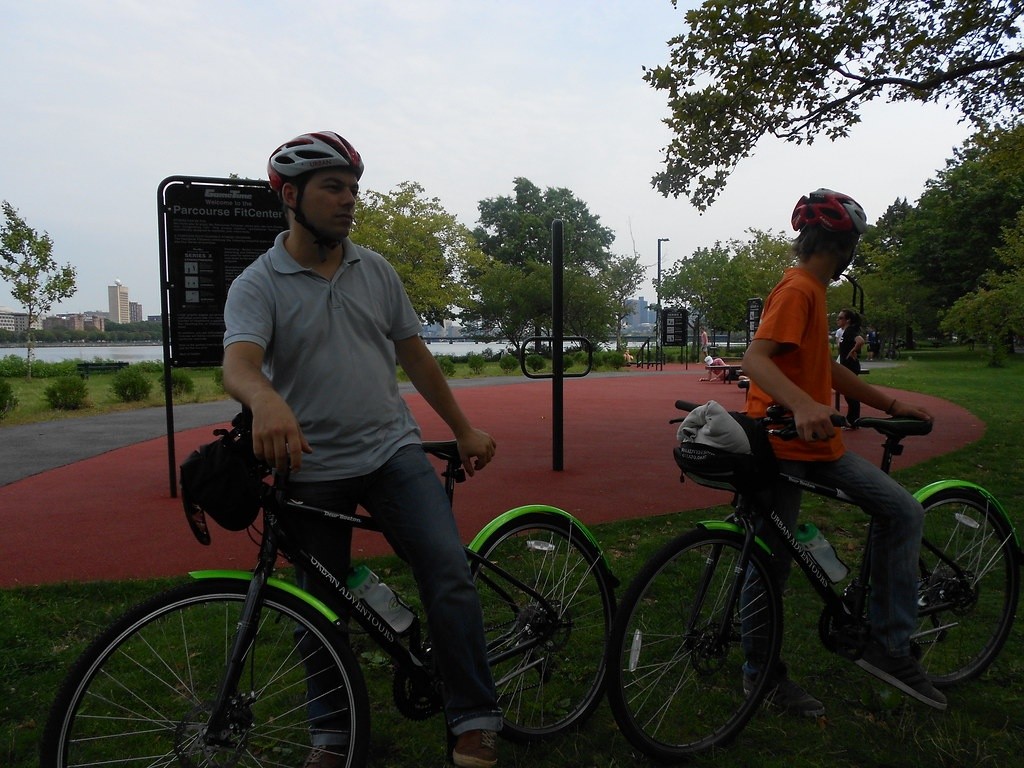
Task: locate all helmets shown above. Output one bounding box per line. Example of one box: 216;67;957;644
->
791;187;868;235
266;130;364;192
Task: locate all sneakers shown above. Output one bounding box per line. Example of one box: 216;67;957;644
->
305;745;345;767
743;672;825;718
855;640;947;710
453;728;499;767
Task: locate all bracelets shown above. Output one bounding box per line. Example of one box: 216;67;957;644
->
884;398;896;414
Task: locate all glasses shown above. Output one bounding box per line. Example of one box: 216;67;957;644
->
837;317;846;320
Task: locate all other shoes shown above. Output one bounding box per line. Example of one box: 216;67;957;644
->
841;420;856;430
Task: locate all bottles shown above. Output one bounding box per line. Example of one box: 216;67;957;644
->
346;566;415;632
798;523;848;583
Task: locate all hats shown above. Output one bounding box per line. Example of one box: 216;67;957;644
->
705;355;714;366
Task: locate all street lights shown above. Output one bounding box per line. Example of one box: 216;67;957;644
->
658;238;669;368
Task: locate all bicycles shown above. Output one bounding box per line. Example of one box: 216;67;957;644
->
609;398;1024;764
882;343;901;360
39;407;622;768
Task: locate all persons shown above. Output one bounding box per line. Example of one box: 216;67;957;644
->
221;131;505;768
739;190;946;716
624;349;634;366
835;307;865;431
835;323;881;361
698;327;708;364
698;356;749;382
